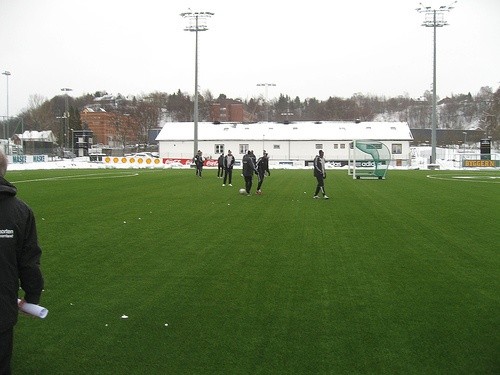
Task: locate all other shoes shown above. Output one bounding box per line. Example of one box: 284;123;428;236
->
314;196;320;199
255;191;262;194
222;184;225;186
228;184;232;187
323;195;329;199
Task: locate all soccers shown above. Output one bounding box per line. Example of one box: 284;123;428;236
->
239;188;246;194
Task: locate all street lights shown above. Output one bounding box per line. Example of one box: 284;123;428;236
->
2;70;12;155
60;87;72;159
415;4;455;171
179;10;215;169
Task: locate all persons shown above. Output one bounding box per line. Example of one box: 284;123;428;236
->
0;152;43;375
217;153;225;178
192;150;205;177
312;149;329;199
241;149;271;197
222;150;235;187
